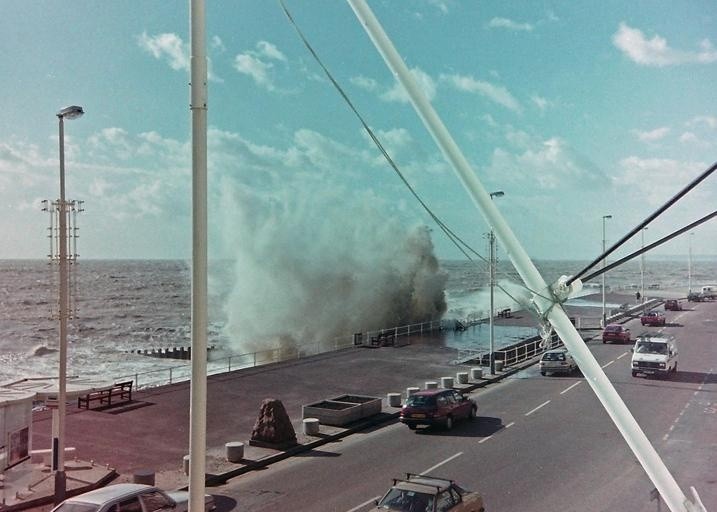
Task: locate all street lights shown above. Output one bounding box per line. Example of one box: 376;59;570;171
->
52;95;83;495
641;226;650;302
602;214;613;327
688;231;695;293
487;188;506;377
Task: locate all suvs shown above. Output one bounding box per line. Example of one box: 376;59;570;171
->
362;470;487;512
397;387;478;432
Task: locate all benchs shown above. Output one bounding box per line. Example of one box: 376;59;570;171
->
372;330;395;347
498;309;511;318
78;380;133;410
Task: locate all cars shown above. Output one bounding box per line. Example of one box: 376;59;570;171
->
600;285;717;345
538;347;576;376
48;480;217;512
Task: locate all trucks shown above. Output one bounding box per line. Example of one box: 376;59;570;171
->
629;330;680;380
